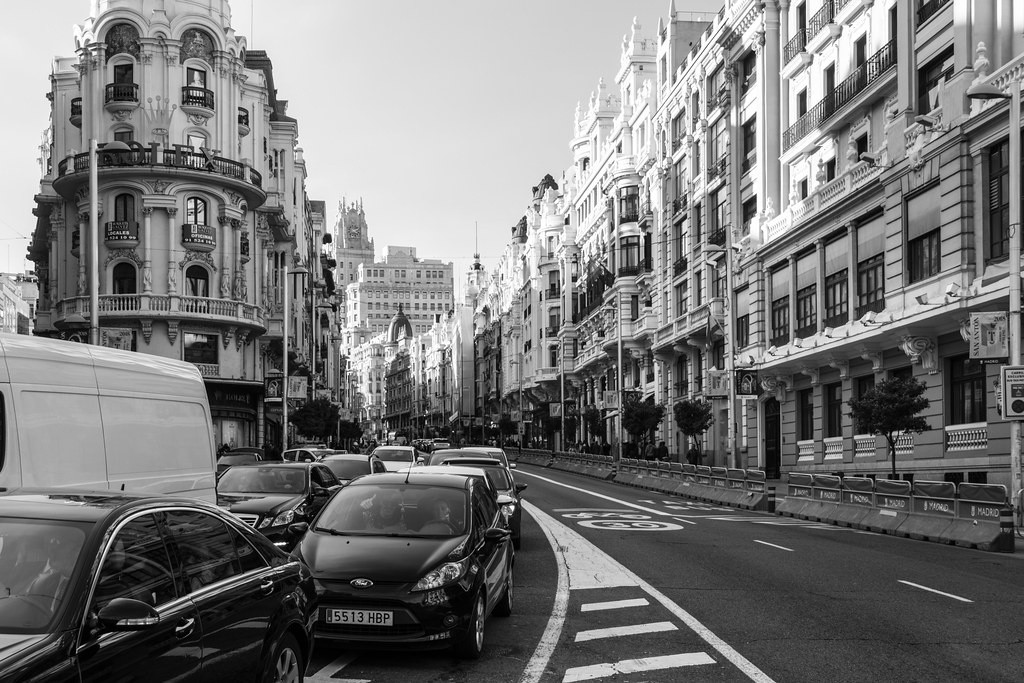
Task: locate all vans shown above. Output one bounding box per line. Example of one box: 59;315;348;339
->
0;329;217;511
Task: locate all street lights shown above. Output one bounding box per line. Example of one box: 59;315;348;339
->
704;225;737;469
967;78;1020;547
283;265;308;455
88;139;133;342
601;292;623;459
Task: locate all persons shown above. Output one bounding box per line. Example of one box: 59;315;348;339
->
424;501;464;532
686;444;699;465
565;439;637;457
485;438;534;447
645;440;669;461
359;494;397;531
268;443;282;461
27;533;132;616
217;443;230;459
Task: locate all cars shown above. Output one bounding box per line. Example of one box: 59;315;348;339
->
0;437;529;683
